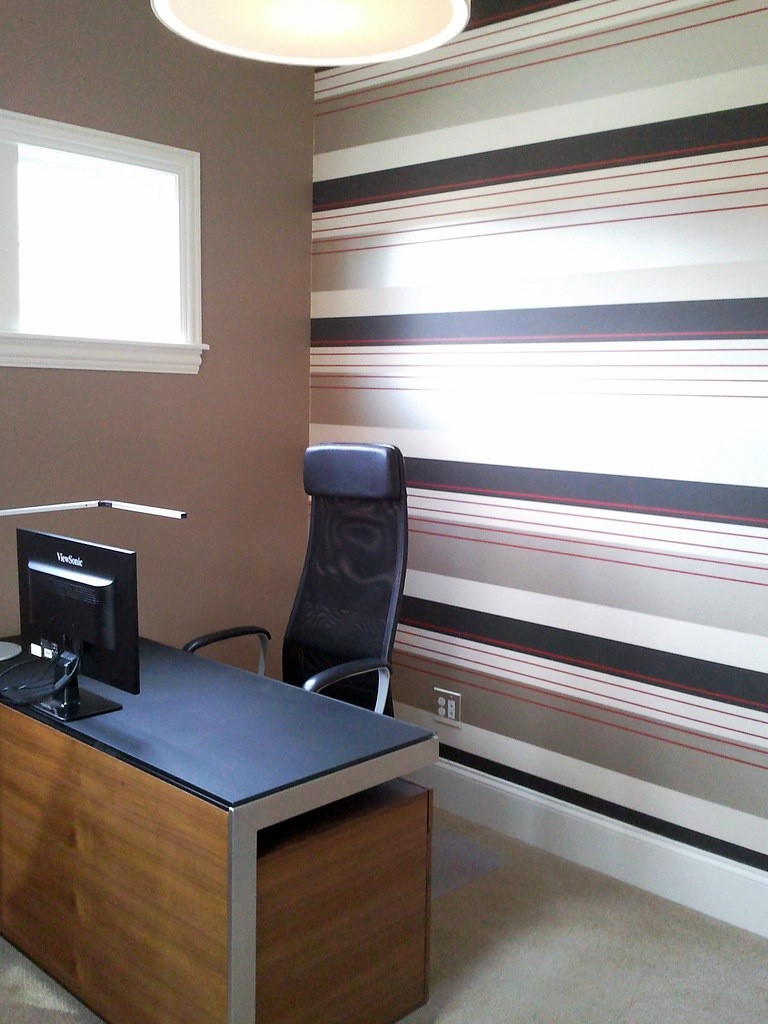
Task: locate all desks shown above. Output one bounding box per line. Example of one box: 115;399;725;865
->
0;634;439;1024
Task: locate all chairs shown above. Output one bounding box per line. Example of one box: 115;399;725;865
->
182;442;407;716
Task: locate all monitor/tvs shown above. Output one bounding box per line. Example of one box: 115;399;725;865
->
15;529;141;722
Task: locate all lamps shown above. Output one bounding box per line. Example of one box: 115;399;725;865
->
151;0;471;71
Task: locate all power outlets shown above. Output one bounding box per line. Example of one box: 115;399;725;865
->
433;687;462;729
